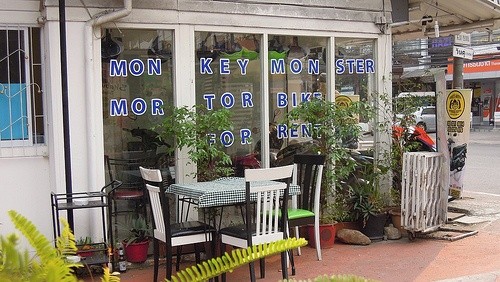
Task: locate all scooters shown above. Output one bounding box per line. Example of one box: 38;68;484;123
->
233;110;321;178
121;127;171;190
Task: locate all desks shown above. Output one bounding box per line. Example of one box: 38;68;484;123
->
164;176;295;282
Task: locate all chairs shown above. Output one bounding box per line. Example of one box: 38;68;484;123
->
104;153;326;282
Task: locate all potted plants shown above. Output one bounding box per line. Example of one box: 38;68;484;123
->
277;93;422;247
78;237;93;255
123;218;153;263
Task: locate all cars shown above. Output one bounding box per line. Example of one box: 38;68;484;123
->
392;106;437;136
